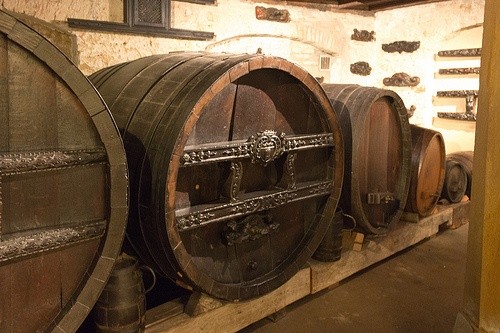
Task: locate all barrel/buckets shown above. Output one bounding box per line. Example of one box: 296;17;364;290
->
0;3;474;333
